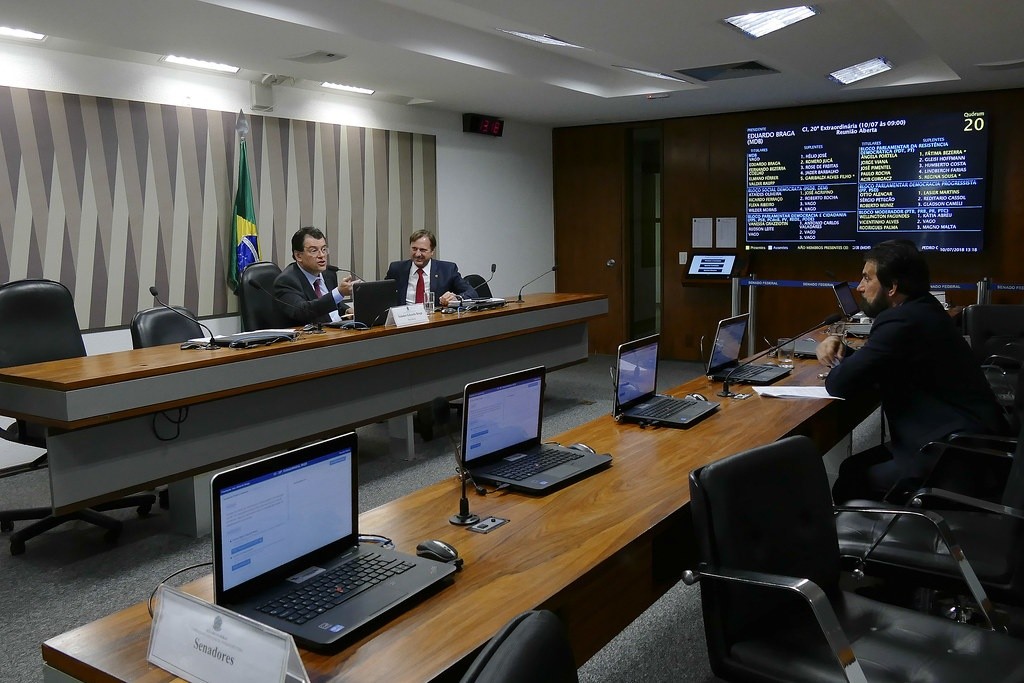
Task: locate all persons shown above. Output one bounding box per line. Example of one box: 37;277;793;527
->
270;226;364;327
383;230;478;306
815;238;1008;511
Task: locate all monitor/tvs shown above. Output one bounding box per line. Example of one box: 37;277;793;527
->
688;254;737;279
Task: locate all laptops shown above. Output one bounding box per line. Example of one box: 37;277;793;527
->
612;333;721;429
832;281;875;323
705;312;792;386
832;323;872;338
929;291;946;308
210;431;457;654
459;364;612;496
448;300;507;309
214;331;300;348
777;339;819;359
323;279;399;329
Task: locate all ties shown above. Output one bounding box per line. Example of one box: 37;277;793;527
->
415;268;426;304
313;278;322;299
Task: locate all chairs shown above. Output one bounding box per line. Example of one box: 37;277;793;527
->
239;261;284;333
460;610;579;683
0;280;155;556
684;305;1023;683
127;307;205;509
464;274;493;298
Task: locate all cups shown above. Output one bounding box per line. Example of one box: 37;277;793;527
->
777;338;795;369
424;292;435;314
830;321;845;342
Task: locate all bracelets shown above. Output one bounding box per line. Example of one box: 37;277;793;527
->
455;295;463;306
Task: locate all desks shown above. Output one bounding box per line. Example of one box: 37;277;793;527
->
40;325;869;683
0;293;609;536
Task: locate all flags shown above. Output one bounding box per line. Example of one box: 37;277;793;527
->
227;140;262;293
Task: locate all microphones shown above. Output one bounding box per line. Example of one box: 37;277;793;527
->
718;313;842;397
515;265;561;303
457;263;496;311
149;286;221;350
326;264;366;282
432;396;480;525
248;279;326;334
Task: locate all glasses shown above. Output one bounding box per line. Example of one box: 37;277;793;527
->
301;245;330;256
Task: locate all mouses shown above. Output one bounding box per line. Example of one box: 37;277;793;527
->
442;307;456;314
804;337;818;343
180;342;201;350
684;392;708;402
303;324;318;331
568;442;596;455
762;361;779;367
416;540;458;563
